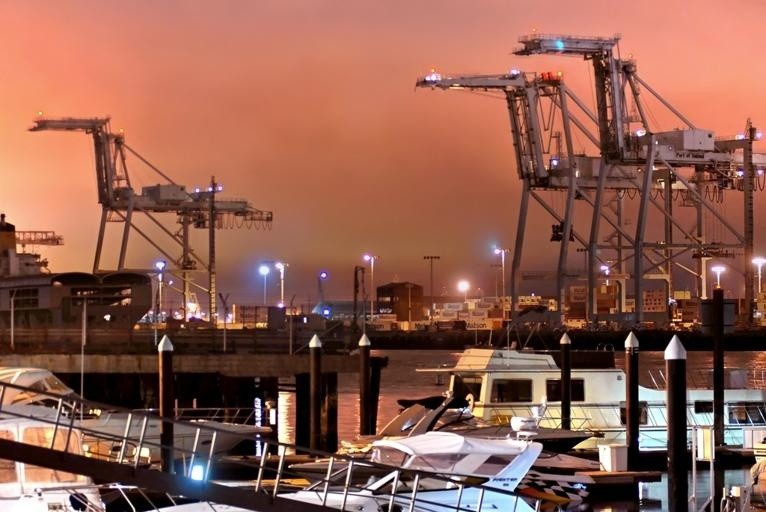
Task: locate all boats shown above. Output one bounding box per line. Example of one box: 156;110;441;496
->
0;347;766;512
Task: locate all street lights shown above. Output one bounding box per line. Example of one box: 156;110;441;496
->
490;248;511;318
751;256;766;294
423;255;441;322
155;261;166;314
601;265;610;287
318;272;328;301
712;265;726;288
364;254;380;319
576;248;589;323
258;263;285;307
458;279;470;303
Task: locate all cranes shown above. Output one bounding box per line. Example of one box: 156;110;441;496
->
3;221;69;251
